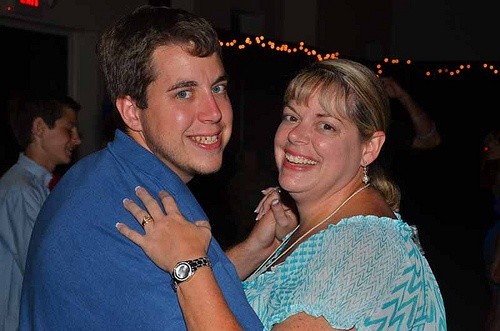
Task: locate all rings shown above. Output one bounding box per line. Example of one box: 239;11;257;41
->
277;186;284;198
141;214;153;227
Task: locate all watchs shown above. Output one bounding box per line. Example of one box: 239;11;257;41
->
169;256;213;292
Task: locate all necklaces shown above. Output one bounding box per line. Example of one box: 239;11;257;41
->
243;180;371;285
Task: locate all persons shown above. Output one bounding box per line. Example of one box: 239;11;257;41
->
225;77;500;331
17;4;283;331
0;91;81;330
114;60;447;331
